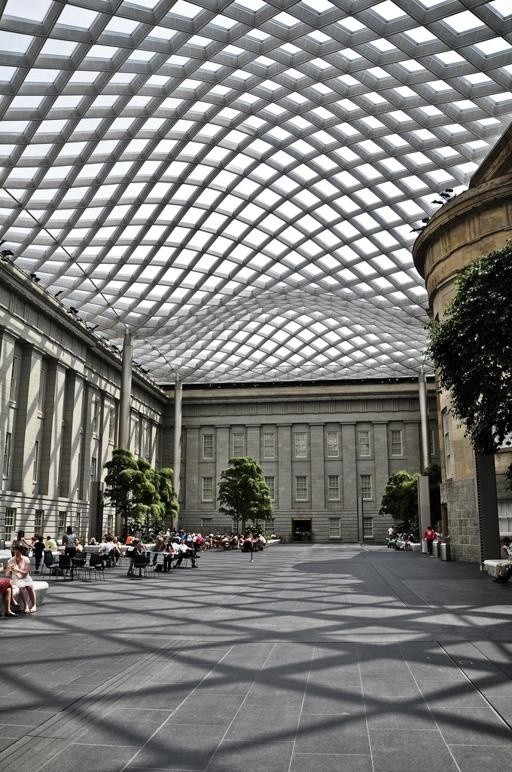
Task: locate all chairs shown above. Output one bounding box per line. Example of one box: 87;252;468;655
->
386;536;421;551
44;529;246;581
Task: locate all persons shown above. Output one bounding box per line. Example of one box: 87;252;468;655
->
6;544;36;613
0;577;20;617
491;536;511;583
423;525;437;555
387;525;417;551
13;526;265;576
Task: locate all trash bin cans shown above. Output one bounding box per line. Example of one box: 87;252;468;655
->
432;541;450;561
422;539;429;553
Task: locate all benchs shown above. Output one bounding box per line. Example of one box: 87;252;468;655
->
483;559;512;576
0;574;49;612
256;537;281;551
